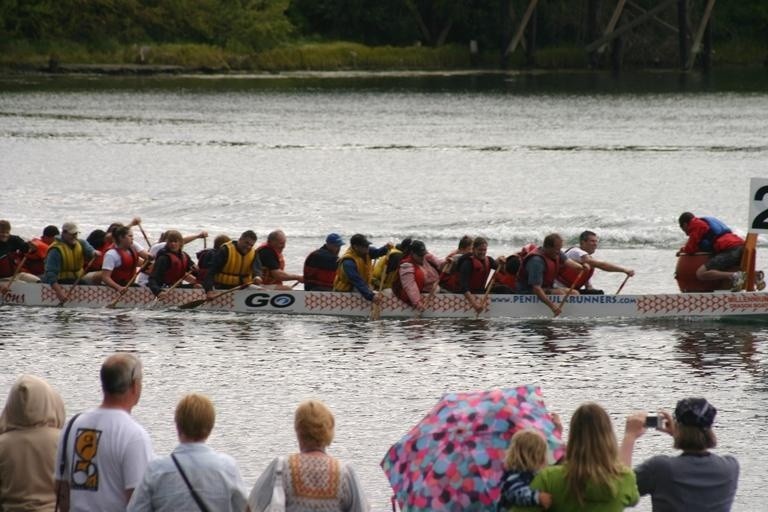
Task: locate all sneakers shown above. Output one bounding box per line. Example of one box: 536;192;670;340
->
731;272;746;292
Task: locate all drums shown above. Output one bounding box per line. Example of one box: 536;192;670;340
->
675;251;716;292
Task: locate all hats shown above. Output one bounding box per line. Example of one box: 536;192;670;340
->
61;222;81;235
411;240;429;257
326;232;346;246
351;233;372;247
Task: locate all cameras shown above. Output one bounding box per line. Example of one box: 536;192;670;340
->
645;414;663;429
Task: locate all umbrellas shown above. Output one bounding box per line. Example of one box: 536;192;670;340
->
380;381;568;512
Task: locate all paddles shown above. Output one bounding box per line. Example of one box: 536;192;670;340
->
371;247;392;321
55;257;96;307
178;281;254;310
105;259;150;308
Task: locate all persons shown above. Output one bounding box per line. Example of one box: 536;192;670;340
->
440;235;474;289
101;226;154;294
333;234;394;319
246;401;371;511
618;398;740;512
509;404;640;512
391;240;454;316
148;231;208;263
497;429;553;512
148;229;199;303
446;237;506;319
125;394;251;512
54;354;154;512
84;217;149;269
0;220;38;296
252;231;304;290
303;234;345;291
0;374;66;512
203;231;258;301
25;225;62;276
43;221;102;303
553;231;635;294
674;210;766;293
372;238;413;291
196;235;231;280
520;234;591;316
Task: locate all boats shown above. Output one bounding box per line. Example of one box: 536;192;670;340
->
0;271;767;323
1;280;767;319
